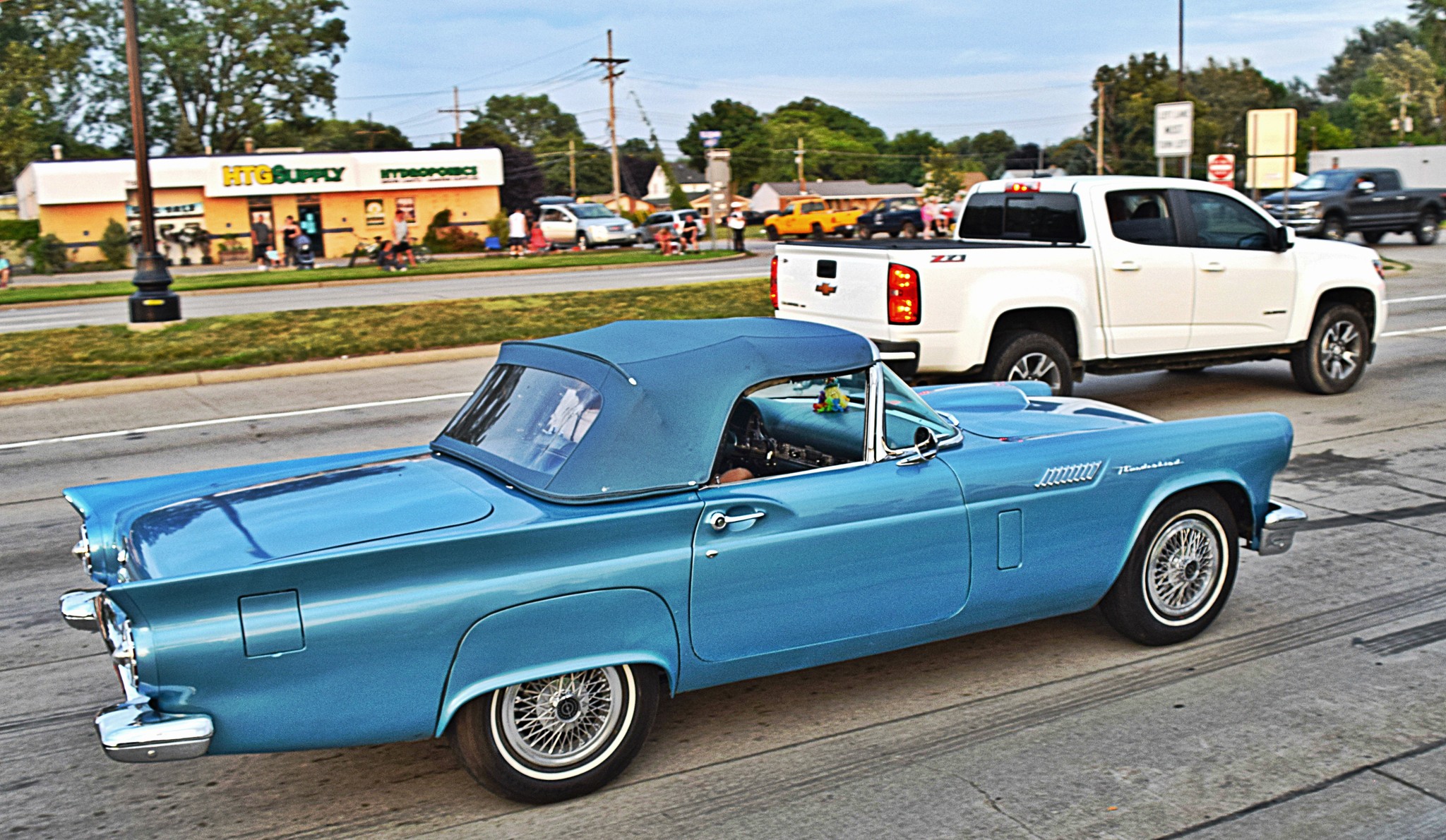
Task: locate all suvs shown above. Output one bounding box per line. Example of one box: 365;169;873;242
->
534;195;638;251
635;210;708;244
998;167;1070;180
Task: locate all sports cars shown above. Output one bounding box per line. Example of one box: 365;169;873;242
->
57;320;1307;808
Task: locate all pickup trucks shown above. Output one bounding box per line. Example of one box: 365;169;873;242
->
1257;168;1446;247
763;198;864;242
768;175;1388;399
856;195;956;241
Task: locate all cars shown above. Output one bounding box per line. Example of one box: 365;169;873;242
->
718;208;779;227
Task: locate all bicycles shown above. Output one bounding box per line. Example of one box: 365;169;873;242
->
408;236;432;265
347;233;377;268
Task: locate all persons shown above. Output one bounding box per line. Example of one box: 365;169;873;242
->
279;215;302;267
710;430;754;485
508;206;562;260
727;201;746;251
680;215;699;255
914;193;957;240
0;251;10;290
375;209;417;273
655;226;672;253
251;214;282;267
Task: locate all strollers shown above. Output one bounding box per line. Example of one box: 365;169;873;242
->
293;234;315;271
257;242;281;269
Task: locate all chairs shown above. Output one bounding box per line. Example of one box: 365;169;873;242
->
1127;201;1166;244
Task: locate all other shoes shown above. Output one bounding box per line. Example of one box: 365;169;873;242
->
257;265;268;271
0;282;8;290
509;252;527;260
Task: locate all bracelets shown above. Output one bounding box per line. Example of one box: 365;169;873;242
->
715;476;720;485
